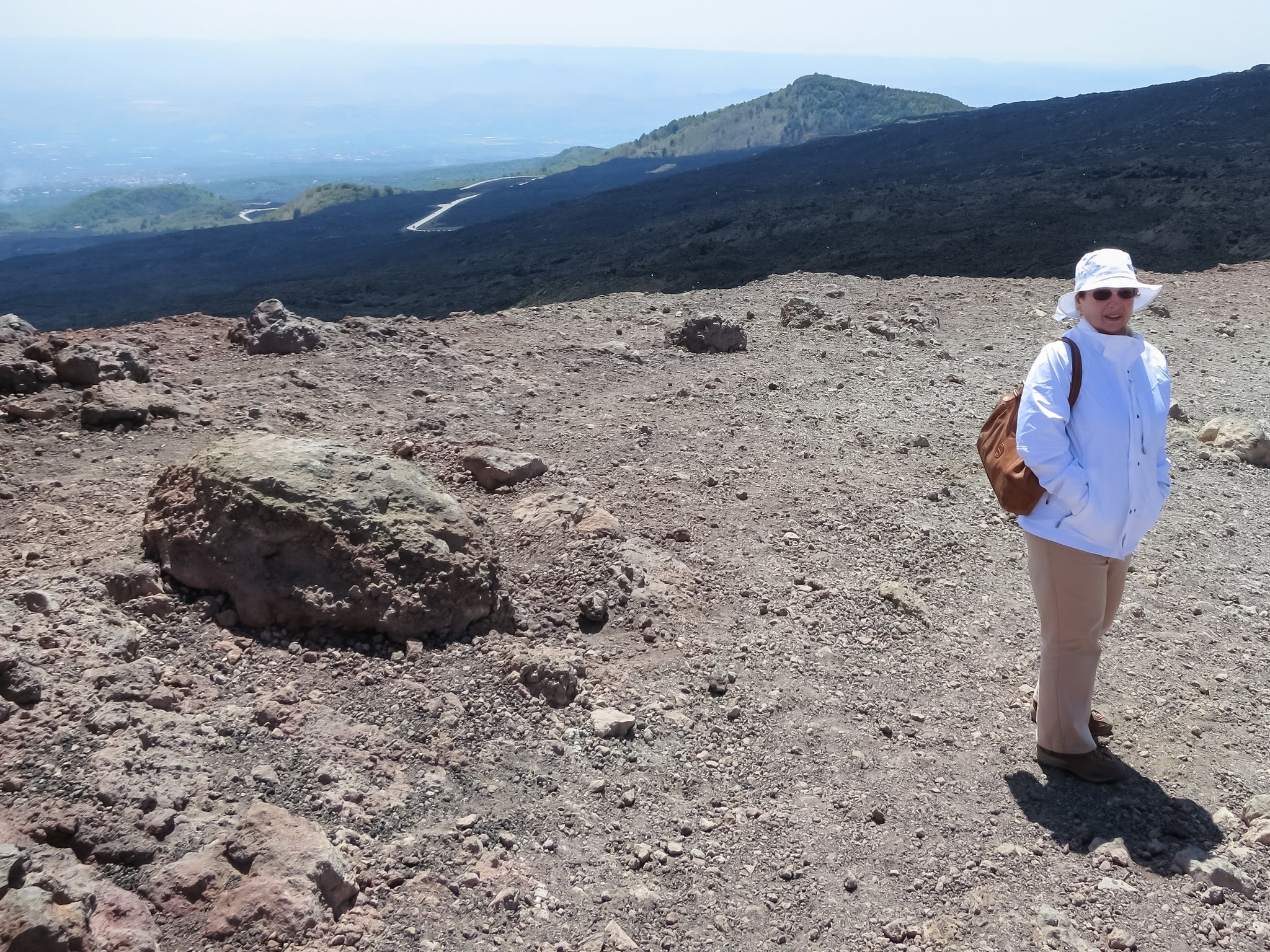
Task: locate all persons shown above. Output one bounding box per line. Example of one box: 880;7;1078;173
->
1015;248;1170;783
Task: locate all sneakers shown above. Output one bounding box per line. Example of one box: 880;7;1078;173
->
1031;698;1113;735
1036;744;1125;781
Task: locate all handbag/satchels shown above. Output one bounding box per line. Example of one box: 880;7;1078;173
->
977;337;1082;515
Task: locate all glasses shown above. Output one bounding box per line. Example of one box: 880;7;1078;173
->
1084;288;1138;301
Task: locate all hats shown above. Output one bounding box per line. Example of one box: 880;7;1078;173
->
1052;248;1163;321
